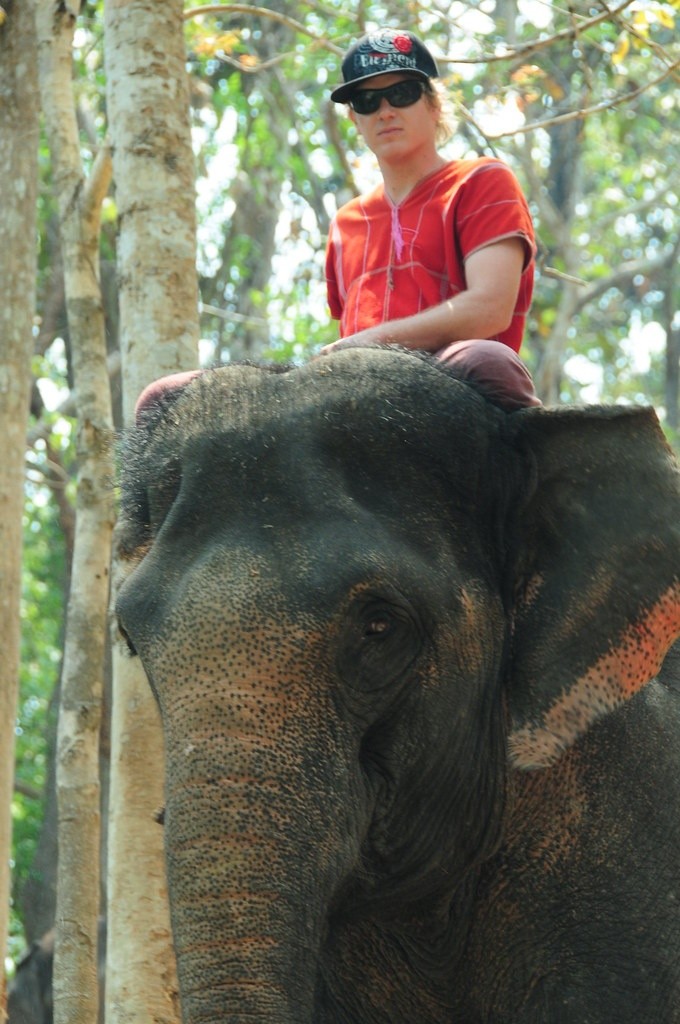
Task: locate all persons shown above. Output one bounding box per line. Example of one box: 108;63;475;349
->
133;28;542;426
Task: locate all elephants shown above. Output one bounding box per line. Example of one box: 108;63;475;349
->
114;344;679;1024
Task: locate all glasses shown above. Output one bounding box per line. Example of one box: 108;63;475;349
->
346;79;429;115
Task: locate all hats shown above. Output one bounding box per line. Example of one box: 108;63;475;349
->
330;30;439;105
